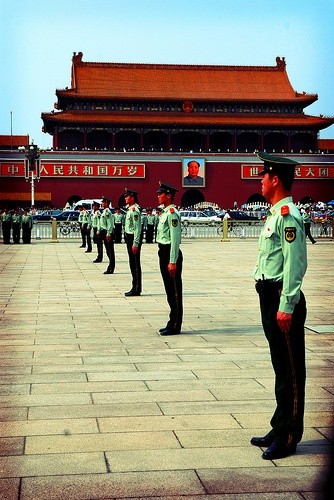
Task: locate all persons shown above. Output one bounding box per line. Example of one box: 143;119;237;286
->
184;161;203;185
295;200;334;244
0;206;37;244
177;204;270;231
249;151;308;461
78;181;183;336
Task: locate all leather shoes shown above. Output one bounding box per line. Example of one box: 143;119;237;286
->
104;270;113;274
80;245;86;247
251;435;273;447
93;258;102;262
262;441;296;459
86;250;91;252
160;327;180;335
125;292;140;296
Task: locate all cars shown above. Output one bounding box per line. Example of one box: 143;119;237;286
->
50;210;80;224
217;211;260;227
32;209;63;224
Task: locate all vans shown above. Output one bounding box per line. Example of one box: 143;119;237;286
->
64;198;111;213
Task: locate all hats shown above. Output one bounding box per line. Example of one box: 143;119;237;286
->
156;180;179;195
257;151;300;175
124;187;140;197
102;196;111;204
23;207;29;212
14;207;19;211
93;201;100;206
305;208;310;211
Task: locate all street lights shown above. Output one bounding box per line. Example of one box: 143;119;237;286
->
18;145;41;208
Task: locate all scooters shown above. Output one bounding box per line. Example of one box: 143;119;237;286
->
59;223;81;236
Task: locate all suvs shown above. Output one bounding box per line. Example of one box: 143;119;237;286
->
178;210;221;227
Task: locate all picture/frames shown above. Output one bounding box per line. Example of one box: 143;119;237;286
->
181;156;205;188
